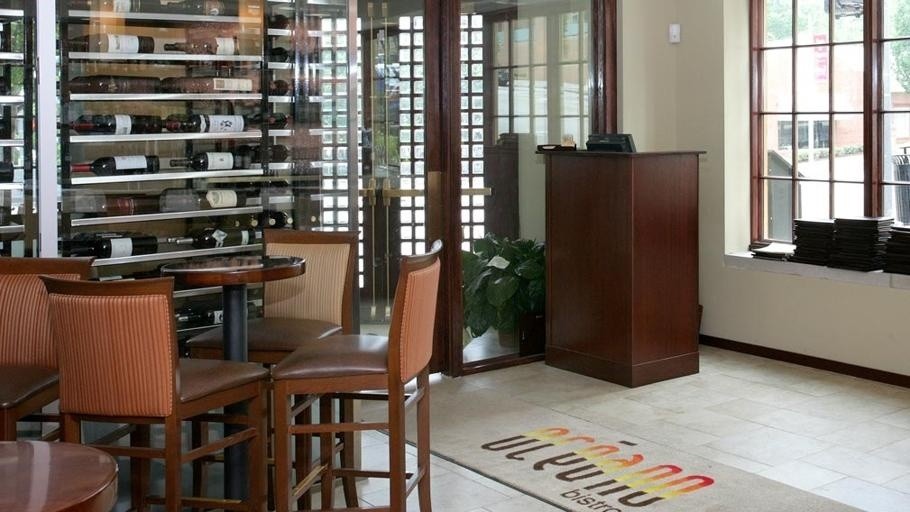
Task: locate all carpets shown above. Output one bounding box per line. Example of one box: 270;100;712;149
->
356;377;867;511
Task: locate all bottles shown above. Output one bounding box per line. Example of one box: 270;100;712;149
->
57;0;295;329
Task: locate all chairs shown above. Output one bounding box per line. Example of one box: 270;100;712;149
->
38;276;271;511
189;230;358;492
0;254;95;440
272;238;442;510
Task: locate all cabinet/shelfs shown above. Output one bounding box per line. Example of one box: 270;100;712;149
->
1;1;357;337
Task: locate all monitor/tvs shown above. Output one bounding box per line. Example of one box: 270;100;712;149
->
587;132;637;153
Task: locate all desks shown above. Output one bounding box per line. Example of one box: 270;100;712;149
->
161;256;307;498
0;437;120;510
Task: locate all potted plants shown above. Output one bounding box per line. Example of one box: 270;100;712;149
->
460;231;550;349
